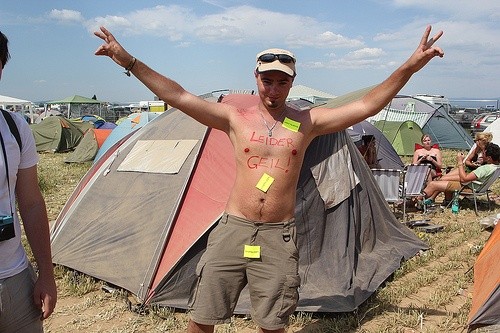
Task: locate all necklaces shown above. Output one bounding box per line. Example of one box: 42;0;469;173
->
259;106;287;137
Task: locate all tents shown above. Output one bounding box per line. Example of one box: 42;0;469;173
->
285;84;476;172
462;118;500;163
31;109;119;163
0;94;34;124
91;113;163;162
50;90;432;314
43;95;101;119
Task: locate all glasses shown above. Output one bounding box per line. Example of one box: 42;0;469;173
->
473;139;479;143
257;53;295;63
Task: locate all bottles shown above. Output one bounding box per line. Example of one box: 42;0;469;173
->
452;189;459;214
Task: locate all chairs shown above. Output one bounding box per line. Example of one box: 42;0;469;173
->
415;143;447;181
446;167;500;216
371;168;407;226
404;164;432;214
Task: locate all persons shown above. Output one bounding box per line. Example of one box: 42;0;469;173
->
467;218;500;327
93;24;444;333
397;144;500;208
465;132;493;169
412;133;442;203
0;30;57;333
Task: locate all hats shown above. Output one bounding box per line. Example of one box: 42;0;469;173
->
255;48;297;77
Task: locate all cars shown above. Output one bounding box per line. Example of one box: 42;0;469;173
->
452;110;500;136
